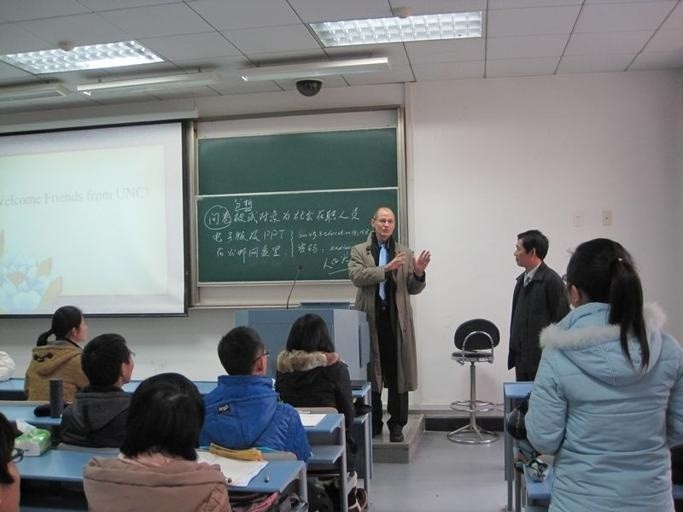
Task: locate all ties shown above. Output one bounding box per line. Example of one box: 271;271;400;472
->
378;244;388;299
524;277;530;287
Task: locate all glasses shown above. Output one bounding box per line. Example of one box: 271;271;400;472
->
5;447;23;465
562;275;569;284
252;351;270;365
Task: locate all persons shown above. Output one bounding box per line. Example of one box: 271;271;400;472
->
0;351;15;380
25;306;88;401
348;207;432;442
197;326;334;511
275;314;360;476
83;373;232;511
507;231;572;382
0;413;24;511
61;333;137;447
507;388;541;473
525;238;683;511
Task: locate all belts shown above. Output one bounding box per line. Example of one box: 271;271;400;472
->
379;305;389;312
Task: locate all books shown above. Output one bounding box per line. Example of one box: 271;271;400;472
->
194;442;268;486
297;412;341;431
350;378;367;390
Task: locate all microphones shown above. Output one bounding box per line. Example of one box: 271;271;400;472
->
286;264;302;309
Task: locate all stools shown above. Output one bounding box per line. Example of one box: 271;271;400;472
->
447;320;500;444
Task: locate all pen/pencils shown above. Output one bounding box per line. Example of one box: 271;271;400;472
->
266;468;270;482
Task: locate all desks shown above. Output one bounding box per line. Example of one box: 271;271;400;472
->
502;380;536;480
7;445;310;511
233;308;370;389
524;465;682;507
296;408;348;510
0;401;66;428
126;380;373;480
1;375;125;394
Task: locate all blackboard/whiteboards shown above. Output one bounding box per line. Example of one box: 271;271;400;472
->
196;126;399;285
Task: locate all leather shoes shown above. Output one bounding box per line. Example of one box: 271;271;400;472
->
390;429;403;442
372;422;382;438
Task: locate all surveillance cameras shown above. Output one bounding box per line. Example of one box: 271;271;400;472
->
295;77;323;98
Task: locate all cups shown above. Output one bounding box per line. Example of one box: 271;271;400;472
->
49;379;63;416
